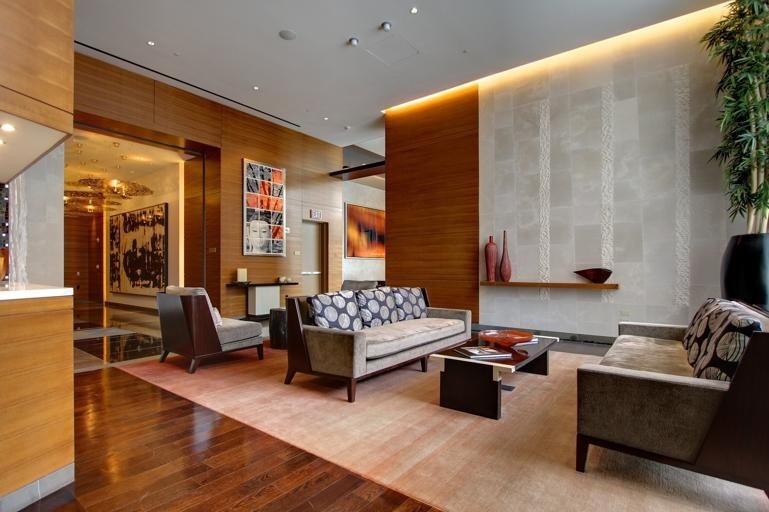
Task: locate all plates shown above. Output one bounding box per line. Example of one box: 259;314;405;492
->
232;280;251;285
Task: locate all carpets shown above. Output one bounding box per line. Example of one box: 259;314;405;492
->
111;351;769;512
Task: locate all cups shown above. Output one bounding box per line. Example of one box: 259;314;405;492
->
277;276;286;283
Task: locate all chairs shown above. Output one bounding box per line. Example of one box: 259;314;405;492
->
155;285;264;374
340;278;387;291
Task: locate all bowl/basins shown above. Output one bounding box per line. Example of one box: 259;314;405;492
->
286;279;292;283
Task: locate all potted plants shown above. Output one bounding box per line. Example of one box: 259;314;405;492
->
699;0;769;316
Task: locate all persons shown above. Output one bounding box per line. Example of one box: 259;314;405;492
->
124;235;162;289
247;213;273;255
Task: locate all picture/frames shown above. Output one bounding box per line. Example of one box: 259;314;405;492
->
243;157;287;257
343;201;386;259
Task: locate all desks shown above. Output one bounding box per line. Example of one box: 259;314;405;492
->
225;281;299;322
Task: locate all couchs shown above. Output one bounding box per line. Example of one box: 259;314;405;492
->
283;285;473;403
576;298;768;494
